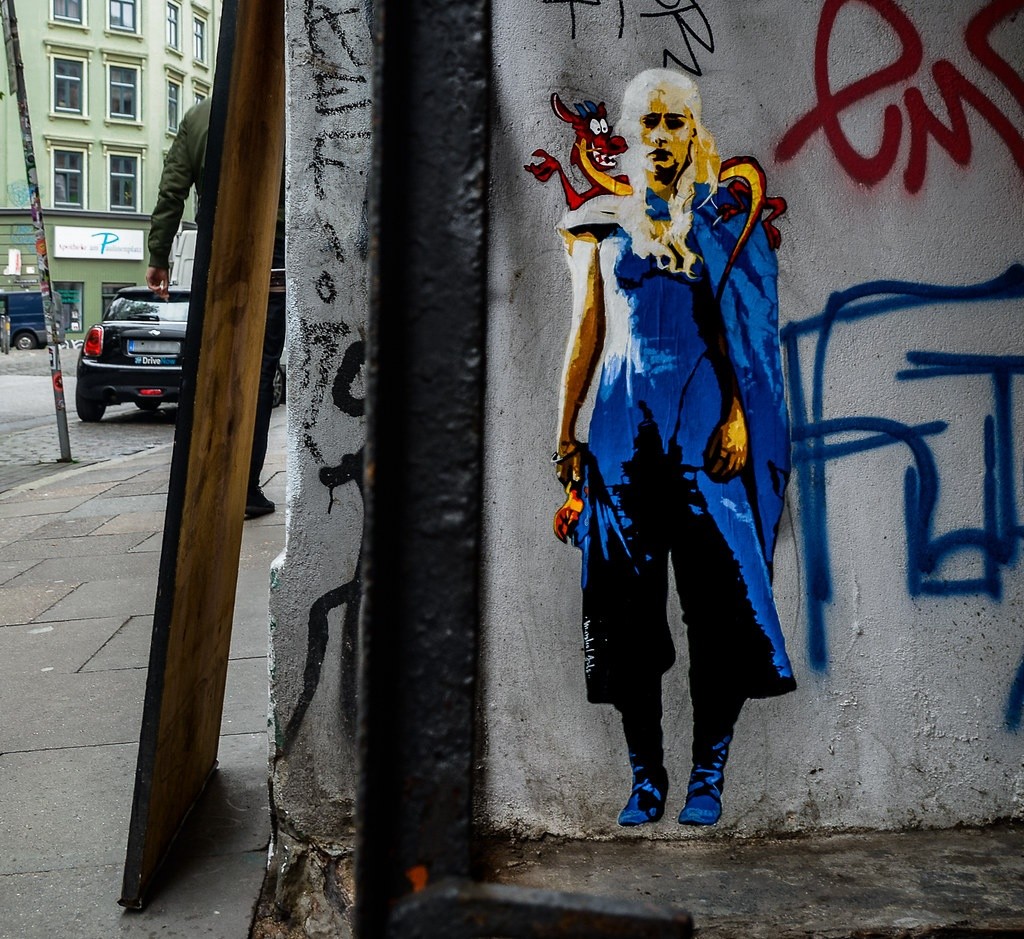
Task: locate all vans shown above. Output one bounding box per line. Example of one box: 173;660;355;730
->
0;290;72;351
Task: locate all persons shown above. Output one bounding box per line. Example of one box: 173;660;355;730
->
145;98;285;520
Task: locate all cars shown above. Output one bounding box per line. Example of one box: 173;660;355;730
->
75;285;284;422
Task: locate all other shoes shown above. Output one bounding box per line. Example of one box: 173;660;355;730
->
244;486;275;514
